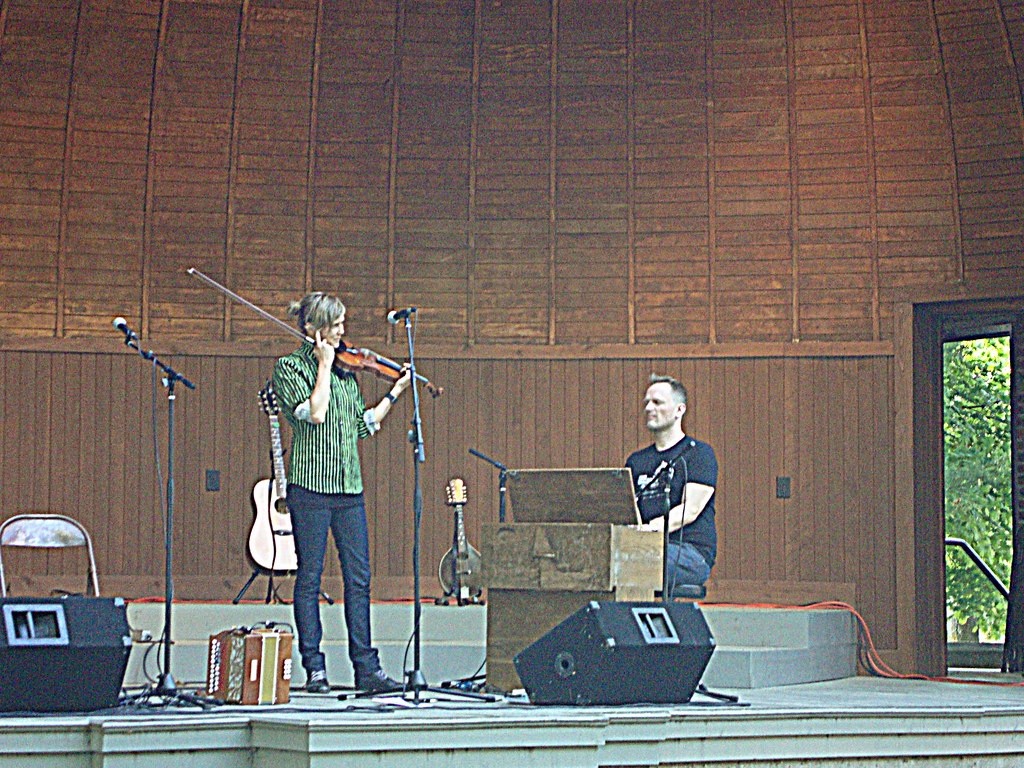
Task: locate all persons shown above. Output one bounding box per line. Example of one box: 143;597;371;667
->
271;291;415;699
625;373;718;602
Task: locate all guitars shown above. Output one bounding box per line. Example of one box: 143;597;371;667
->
245;387;299;573
437;477;483;599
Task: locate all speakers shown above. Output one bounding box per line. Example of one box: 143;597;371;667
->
514;600;717;709
0;595;132;713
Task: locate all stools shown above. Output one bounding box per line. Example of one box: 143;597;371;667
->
654;584;707;599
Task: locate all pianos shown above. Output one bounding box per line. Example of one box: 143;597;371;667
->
478;520;668;696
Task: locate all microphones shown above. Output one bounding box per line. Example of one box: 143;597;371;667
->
386;308;416;324
112;317;138;340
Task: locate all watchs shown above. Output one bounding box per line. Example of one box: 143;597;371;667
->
385;392;398;404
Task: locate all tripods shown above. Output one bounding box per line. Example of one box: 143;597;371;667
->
117;345;224;709
338;317;498;705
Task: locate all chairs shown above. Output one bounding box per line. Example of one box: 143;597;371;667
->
0;514;100;597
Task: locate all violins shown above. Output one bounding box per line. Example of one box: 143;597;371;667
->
334;339;446;399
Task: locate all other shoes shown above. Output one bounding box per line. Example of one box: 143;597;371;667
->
354;671;396;690
306;670;330;694
